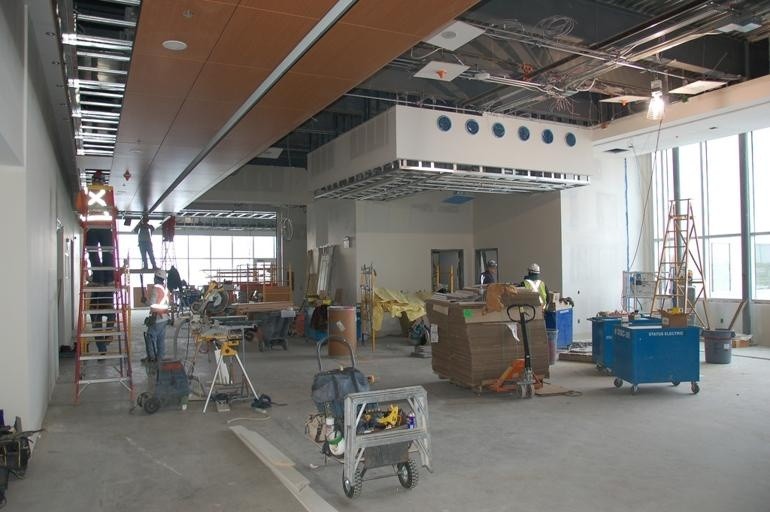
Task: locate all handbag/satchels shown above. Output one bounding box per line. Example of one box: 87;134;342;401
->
144;316;155;328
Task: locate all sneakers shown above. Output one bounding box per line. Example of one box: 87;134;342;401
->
140;357;155;363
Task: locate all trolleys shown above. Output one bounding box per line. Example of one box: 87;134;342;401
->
316;335;373;464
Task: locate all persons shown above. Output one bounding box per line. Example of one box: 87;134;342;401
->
136;218;158;269
515;263;550;327
75;171;119;287
139;269;168;363
478;259;497;284
84;258;129;361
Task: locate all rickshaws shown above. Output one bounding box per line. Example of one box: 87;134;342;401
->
257;306;296;351
481;302;543;398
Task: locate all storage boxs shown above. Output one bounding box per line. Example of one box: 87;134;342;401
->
732;339;750;348
657;307;689;330
548;291;560;311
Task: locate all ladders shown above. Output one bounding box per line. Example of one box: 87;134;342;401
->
649;199;710;331
74;210;134;405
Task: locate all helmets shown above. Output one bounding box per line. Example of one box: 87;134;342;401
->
154;270;169;280
487;260;497;266
529;263;541;274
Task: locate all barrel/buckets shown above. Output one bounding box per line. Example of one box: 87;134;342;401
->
328;305;356;356
704;327;734;364
